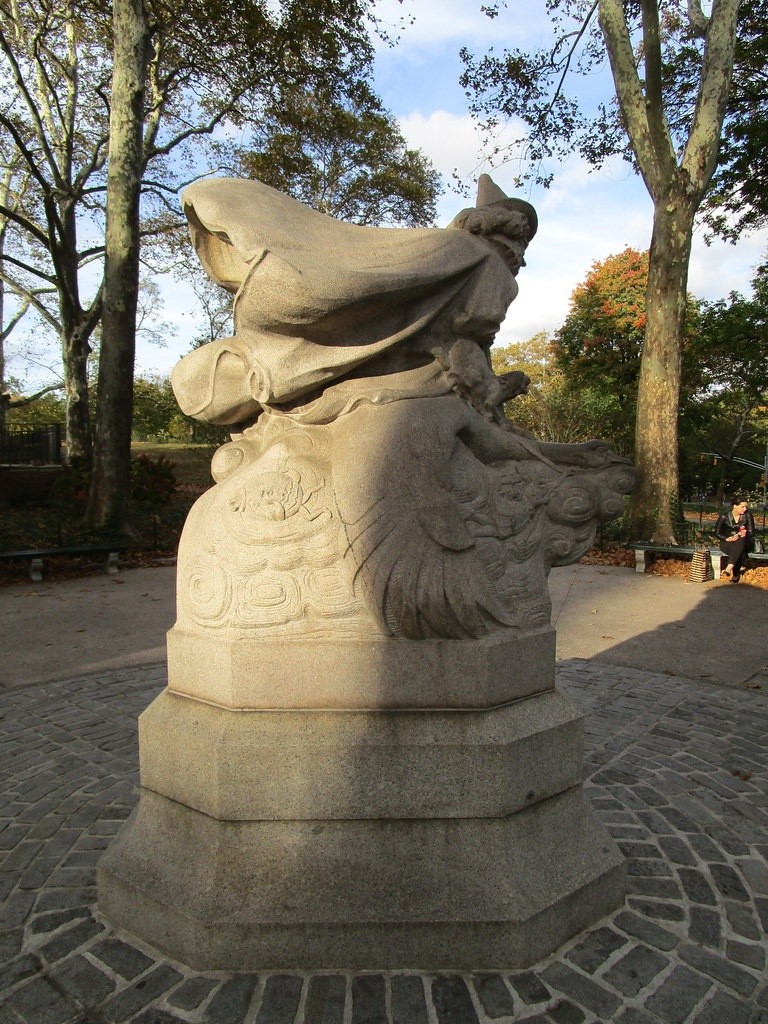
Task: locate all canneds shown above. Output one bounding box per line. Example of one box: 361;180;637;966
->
739;525;746;536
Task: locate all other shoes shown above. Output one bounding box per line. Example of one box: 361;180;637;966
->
722;569;740;583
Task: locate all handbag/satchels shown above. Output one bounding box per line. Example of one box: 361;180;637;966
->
752;539;765;554
688;542;714;582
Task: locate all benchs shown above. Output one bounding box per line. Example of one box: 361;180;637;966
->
627;542;768;580
0;541;130;582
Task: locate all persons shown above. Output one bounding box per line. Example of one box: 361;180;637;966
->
714;495;756;583
